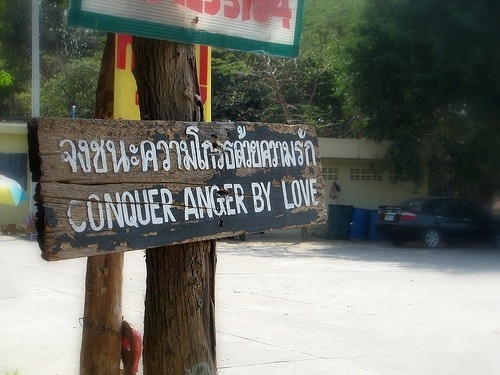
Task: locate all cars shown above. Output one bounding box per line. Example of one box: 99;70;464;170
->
375;196;500;250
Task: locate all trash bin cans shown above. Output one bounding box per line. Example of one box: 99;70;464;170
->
367;209;385;242
327;204;354;241
348;207;370;242
